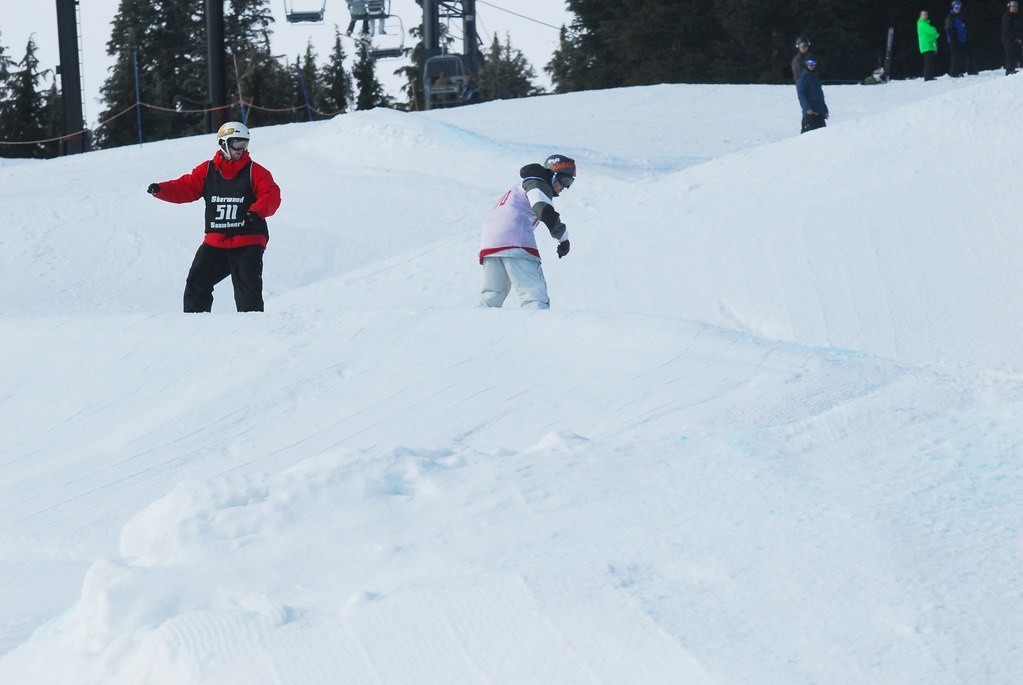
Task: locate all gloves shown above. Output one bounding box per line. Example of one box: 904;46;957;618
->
147;183;159;195
245;211;260;227
556;240;569;258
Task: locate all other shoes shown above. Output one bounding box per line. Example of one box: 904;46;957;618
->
1006;71;1018;75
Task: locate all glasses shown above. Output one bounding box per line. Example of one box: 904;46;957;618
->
798;42;807;47
953;4;960;9
229;140;248;151
557;173;573;188
805;60;816;66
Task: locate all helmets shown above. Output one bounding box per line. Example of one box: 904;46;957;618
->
544;155;575;179
795;36;810;49
951;1;962;7
1007;1;1017;8
216;121;250;144
803;54;817;64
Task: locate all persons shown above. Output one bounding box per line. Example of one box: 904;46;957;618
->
1001;0;1023;76
433;71;454;101
916;10;943;81
347;0;386;35
478;154;576;309
791;34;816;87
147;121;281;313
944;0;967;77
796;54;829;135
